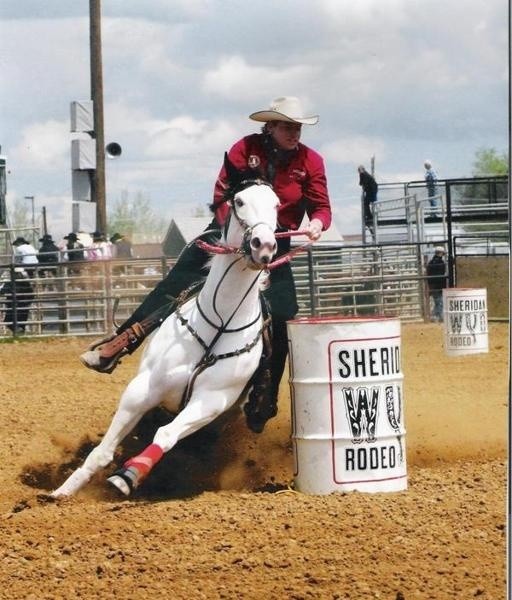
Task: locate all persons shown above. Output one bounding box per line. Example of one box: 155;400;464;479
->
0;266;35;334
424;160;439;206
427;247;447;322
83;97;332;433
11;230;133;280
358;166;378;218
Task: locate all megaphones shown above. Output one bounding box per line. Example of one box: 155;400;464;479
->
105;142;121;159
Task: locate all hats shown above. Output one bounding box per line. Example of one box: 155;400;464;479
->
89;230;105;236
249;96;319;127
38;235;55;244
63;233;78;240
11;237;32;245
436;246;447;254
109;233;123;240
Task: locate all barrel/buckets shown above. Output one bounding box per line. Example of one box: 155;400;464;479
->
439;287;493;356
285;307;410;496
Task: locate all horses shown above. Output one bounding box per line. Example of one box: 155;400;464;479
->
34;150;283;505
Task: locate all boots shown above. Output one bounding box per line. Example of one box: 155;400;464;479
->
80;332;129;374
246;384;278;433
